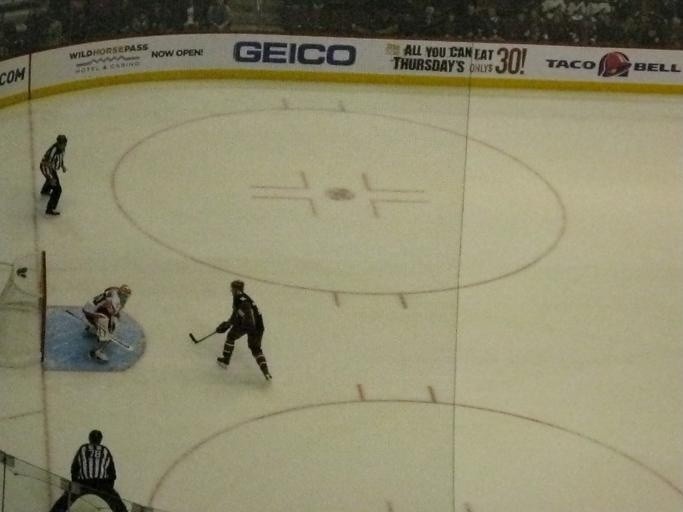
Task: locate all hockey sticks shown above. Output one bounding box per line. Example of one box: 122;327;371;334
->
190;331;218;343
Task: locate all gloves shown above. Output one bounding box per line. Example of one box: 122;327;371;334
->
216;322;230;333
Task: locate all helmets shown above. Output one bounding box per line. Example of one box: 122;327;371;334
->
231;281;244;293
89;430;102;444
119;285;130;298
57;135;67;150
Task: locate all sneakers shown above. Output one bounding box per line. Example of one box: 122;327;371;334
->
94;350;107;362
263;370;271;379
217;357;229;365
41;190;50;195
45;208;59;216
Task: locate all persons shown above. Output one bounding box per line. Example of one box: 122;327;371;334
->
216;281;272;386
83;283;132;361
39;134;69;215
0;0;683;66
50;430;127;512
